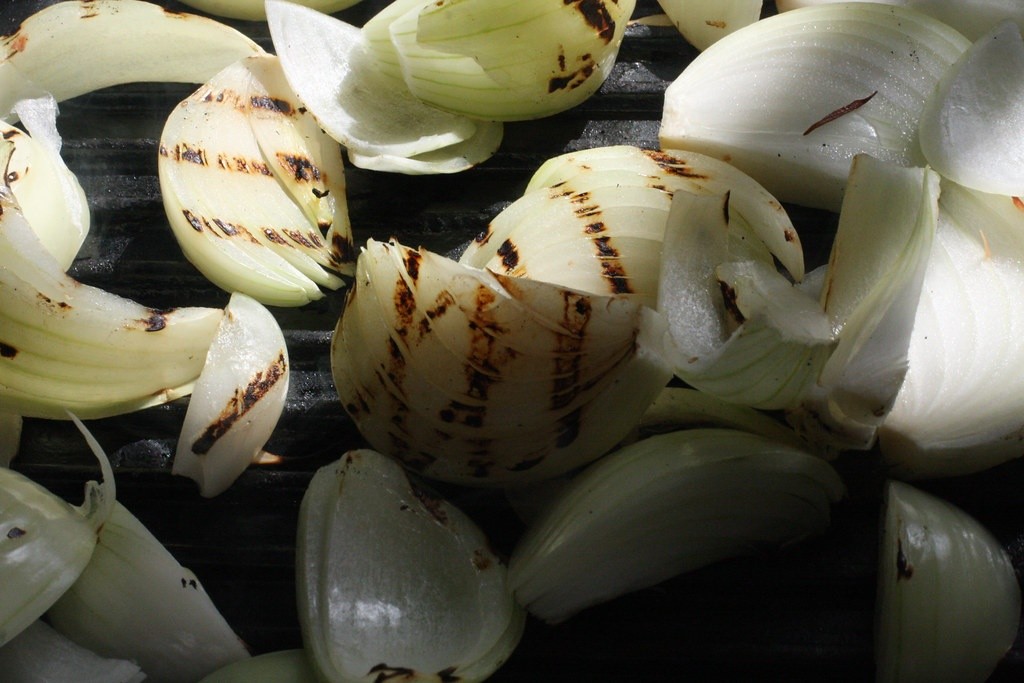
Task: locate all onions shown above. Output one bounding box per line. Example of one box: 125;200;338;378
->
0;0;1024;683
